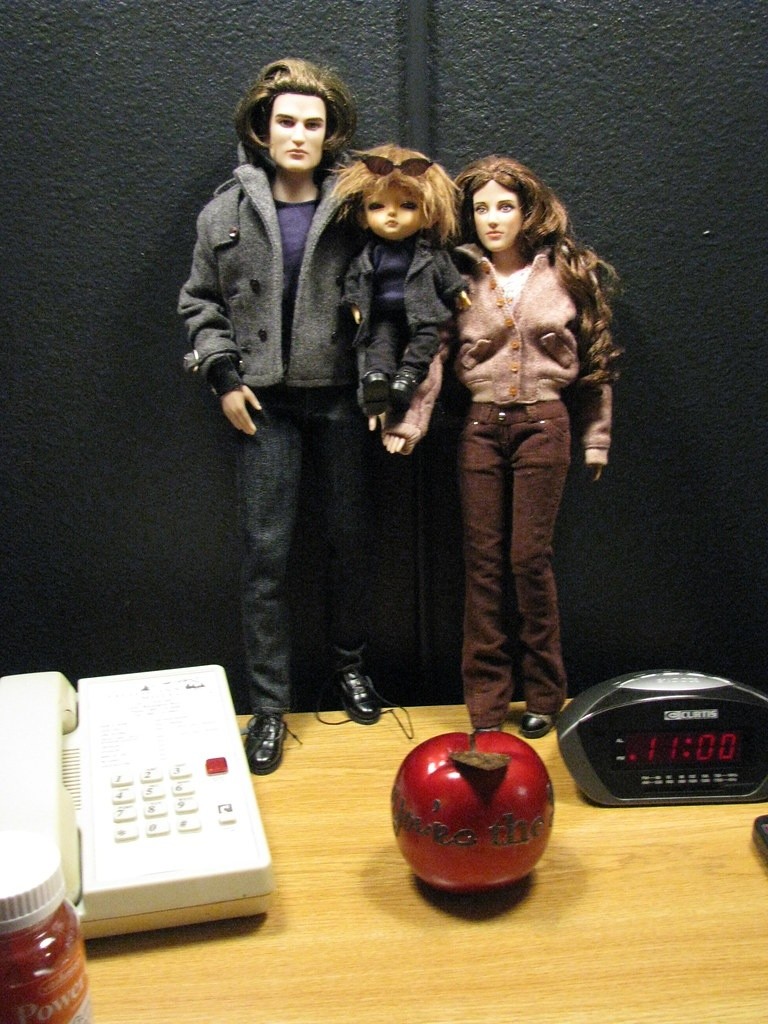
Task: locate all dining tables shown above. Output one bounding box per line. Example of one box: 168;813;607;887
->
84;695;768;1022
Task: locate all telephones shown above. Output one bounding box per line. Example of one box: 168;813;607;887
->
1;663;274;940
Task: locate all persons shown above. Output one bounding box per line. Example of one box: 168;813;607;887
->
329;142;475;412
381;154;624;740
176;56;397;780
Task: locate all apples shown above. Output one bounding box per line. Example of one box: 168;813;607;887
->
392;733;556;898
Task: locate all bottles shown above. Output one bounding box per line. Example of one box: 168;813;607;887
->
0;826;92;1024
